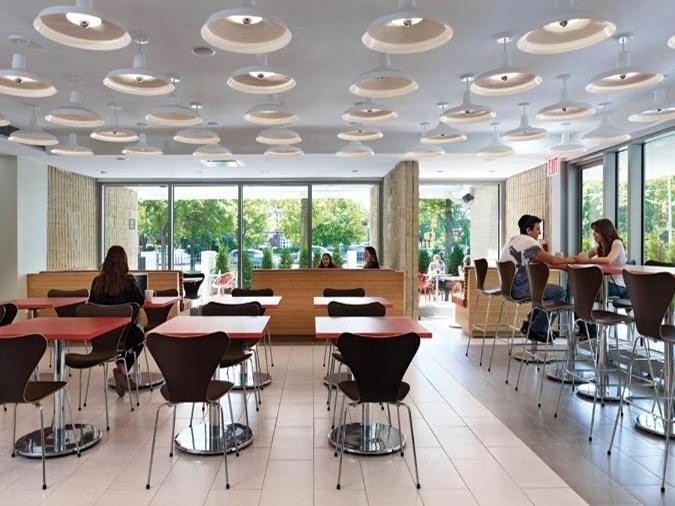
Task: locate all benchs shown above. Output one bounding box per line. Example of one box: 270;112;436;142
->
39;270;193;315
452;267;560;337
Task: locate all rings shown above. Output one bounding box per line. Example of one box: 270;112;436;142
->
574;256;576;259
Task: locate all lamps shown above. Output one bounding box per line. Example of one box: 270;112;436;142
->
190;123;232;162
226;54;297;96
461;185;478;205
336;141;375;157
476;122;513;158
668;36;675;51
469;33;541;97
501;101;550;143
419;102;466;144
145;75;202;127
8;101;60;146
45;81;104;128
517;0;618;56
199;0;292;54
242;97;298;125
1;111;11;127
50;133;94;156
586;33;667;96
32;0;132;51
439;75;497;124
88;103;139;143
349;54;421;100
337;128;384;141
580;103;630;146
121;130;165;156
172;104;221;146
102;35;176;98
547;120;587;156
256;126;303;145
264;146;305;157
406;122;444;157
341;99;398;125
361;0;453;56
629;86;675;122
537;72;597;122
0;35;57;98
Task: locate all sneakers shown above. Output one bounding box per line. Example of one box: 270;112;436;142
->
575;328;584;336
578;330;601;342
528;328;560;343
520;320;529;335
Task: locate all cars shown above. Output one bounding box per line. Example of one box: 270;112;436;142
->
144;243;376;269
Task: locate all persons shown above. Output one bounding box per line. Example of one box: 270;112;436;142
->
499;214;570;343
460;248;471;272
569;218;628;344
362;246;379;268
318;253;338;268
428;254;452;301
87;245;146;397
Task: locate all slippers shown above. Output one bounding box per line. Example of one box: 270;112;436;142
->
117;369;136;391
113;368;125;398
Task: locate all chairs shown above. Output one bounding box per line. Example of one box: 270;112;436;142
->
322;288;367;367
326;301;395;435
1;303;18;326
607;269;674;492
553;265;636;443
144;330;240;491
452;273;465;298
183;273;205;314
515;262;608;411
189;303;261;428
217;301;264;405
417;270;452;303
465;257;513;369
144;288;178;332
0;333;82;489
212;271;235;297
334;332;422;490
487;260;543;388
66;302;134;430
231;288;274;365
611;259;674;415
47;289;89;317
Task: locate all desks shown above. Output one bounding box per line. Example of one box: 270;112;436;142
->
508;262;675;443
1;297;90;316
141;296;183;308
312;297;395;387
144;316;271;455
0;316;132;458
214;293;282;389
183;278;203;283
314;316;431;457
